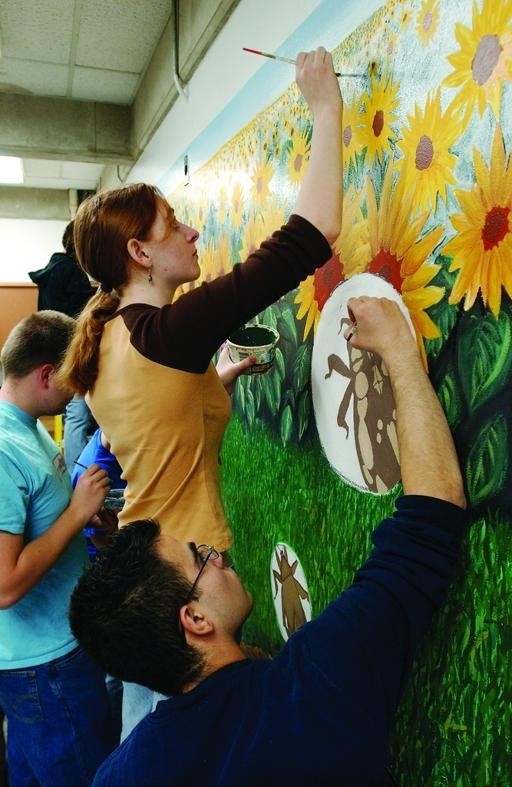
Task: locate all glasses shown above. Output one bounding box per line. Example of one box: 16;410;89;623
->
192;541;218;597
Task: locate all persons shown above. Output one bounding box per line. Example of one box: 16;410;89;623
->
1;310;114;784
71;424;128;565
54;44;344;742
70;295;467;784
28;215;97;480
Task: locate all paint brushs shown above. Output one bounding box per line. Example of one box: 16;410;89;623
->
347;323;357;341
242;48;340;76
74;462;88;469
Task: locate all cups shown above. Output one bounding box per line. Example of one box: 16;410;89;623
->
226;323;281;376
